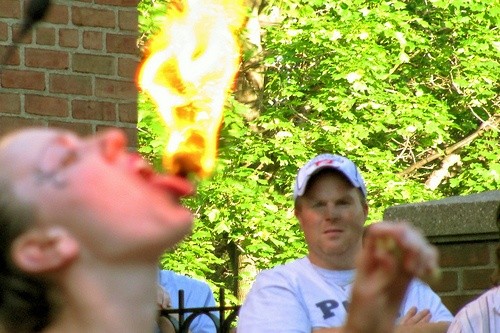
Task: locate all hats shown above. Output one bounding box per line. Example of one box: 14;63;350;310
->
294;153;368;197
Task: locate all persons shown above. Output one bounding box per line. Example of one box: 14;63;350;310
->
446;205;500;333
0;124;418;332
155;265;220;333
238;155;456;333
